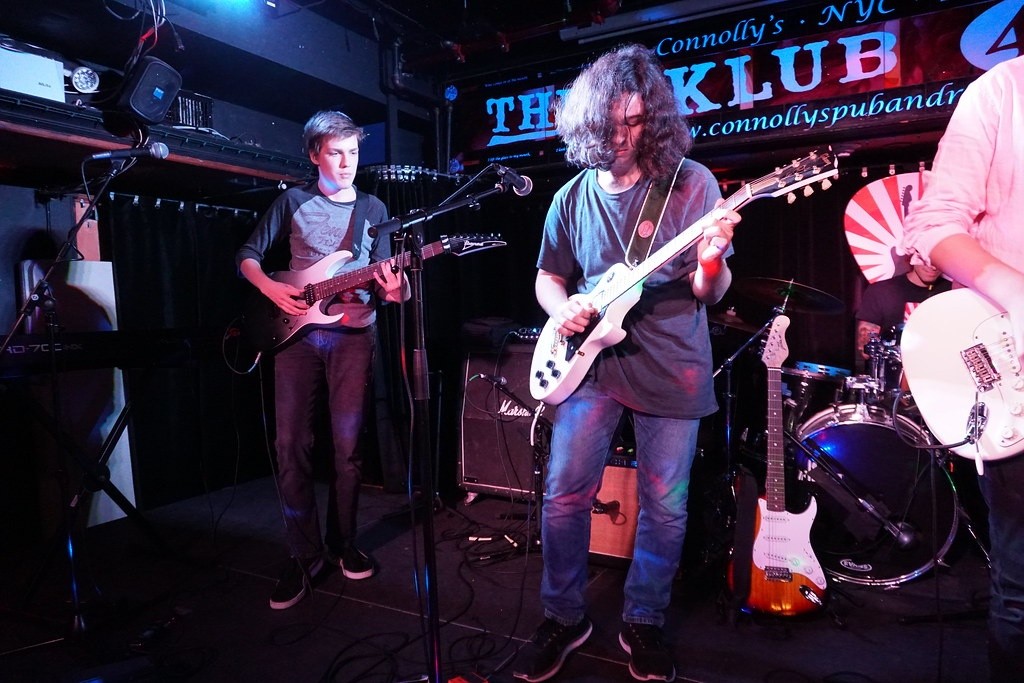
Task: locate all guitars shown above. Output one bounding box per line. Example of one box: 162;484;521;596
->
239;231;510;357
725;315;830;618
898;286;1024;462
527;143;843;407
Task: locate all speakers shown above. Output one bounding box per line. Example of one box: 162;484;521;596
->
458;345;559;502
586;456;643;569
119;56;182;124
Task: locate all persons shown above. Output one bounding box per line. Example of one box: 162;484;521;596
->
905;53;1024;683
512;44;742;683
853;260;968;388
234;110;411;609
0;229;114;608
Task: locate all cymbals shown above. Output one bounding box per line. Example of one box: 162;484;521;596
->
733;274;849;317
706;313;767;335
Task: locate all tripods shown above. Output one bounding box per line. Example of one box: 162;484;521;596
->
0;159;164;661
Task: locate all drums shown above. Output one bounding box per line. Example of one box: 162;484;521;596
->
792;403;961;587
779;367;834;418
839;375;887;404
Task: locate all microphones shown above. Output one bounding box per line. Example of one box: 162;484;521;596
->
91;142;169;160
478;373;507;386
893;522;918;550
490;163;532;196
593;502;619;514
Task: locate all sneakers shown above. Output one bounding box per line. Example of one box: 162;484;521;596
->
325;541;373;579
512;618;593;683
270;554;323;610
619;622;676;683
